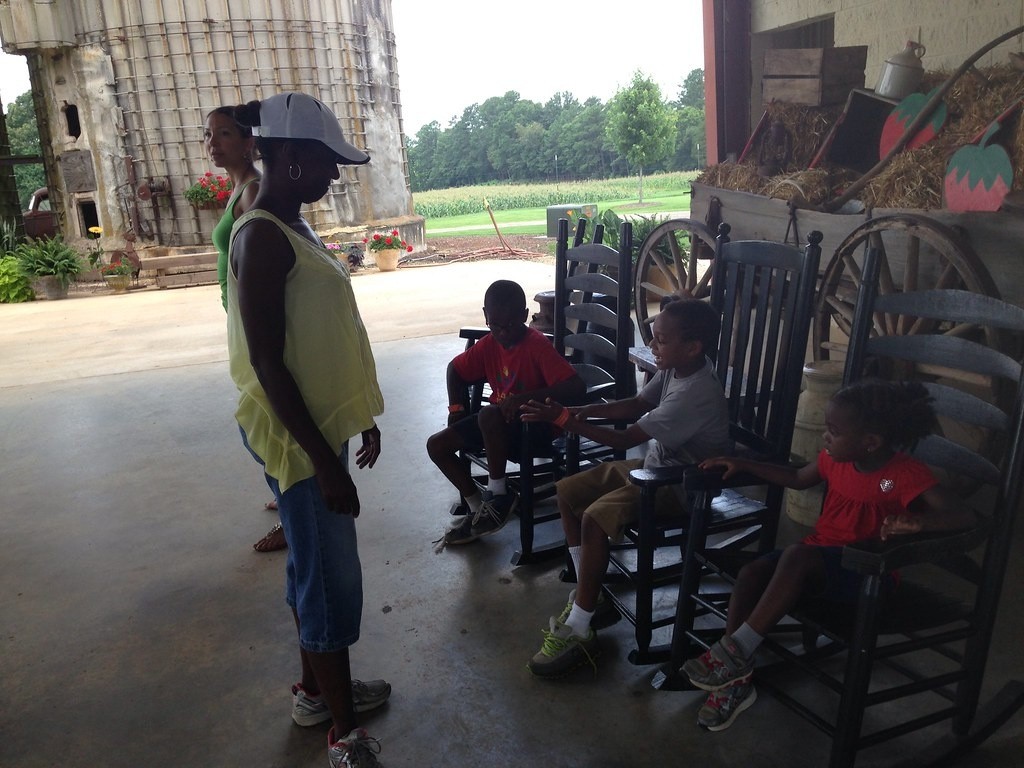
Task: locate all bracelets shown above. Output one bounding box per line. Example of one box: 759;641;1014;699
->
448;403;466;412
553;406;571;428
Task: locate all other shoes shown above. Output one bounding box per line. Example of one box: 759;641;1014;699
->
264;498;279;511
252;522;288;551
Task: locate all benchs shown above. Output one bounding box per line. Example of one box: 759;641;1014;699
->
630;345;776;428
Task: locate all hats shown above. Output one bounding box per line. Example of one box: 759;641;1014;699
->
250;93;372;164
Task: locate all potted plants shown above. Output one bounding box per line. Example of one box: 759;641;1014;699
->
13;234;83;300
622;211;689;302
344;243;366;272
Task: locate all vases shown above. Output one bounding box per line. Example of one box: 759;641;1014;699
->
103;274;132;295
334;254;349;267
601;265;635;289
197;200;227;209
374;249;400;271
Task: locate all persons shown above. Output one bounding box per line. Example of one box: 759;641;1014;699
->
228;92;392;768
680;379;982;732
526;298;733;678
203;105;287;551
427;280;586;545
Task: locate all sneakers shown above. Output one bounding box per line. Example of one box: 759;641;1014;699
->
679;635;755;691
327;725;383;768
444;486;520;544
555;590;623;630
696;683;756;731
289;673;391;727
524;615;603;679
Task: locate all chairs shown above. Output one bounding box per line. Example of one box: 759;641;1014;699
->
451;222;1024;767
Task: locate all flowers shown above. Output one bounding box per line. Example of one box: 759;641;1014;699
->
323;235;348;254
86;227;134;276
363;229;414;252
183;172;231;207
547;206;632;267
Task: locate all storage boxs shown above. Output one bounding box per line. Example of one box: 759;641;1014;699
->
760;45;869;107
